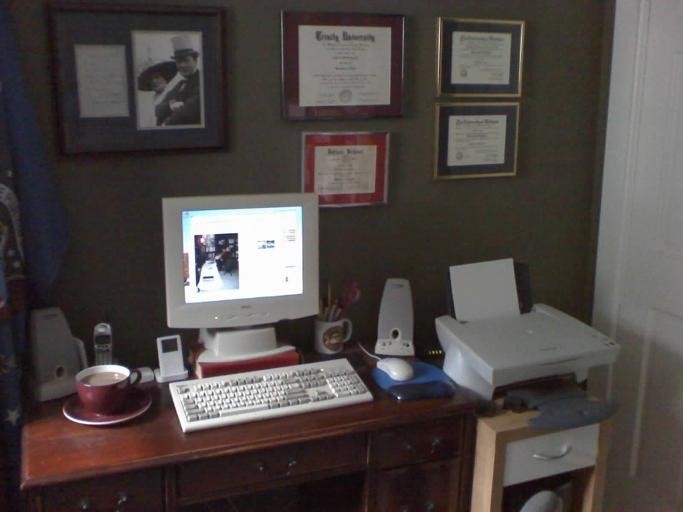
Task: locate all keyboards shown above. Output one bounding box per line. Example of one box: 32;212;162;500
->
169;358;375;434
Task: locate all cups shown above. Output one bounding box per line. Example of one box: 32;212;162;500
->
310;317;353;356
73;364;141;414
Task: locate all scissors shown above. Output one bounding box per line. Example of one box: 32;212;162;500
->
335;279;362;321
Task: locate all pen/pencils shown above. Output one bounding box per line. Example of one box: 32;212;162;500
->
319;283;342;322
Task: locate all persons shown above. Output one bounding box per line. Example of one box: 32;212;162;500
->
137;32;201;126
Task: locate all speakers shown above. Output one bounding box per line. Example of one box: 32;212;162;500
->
375;278;415;355
31;307;80;403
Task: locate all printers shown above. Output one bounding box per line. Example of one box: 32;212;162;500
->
436;260;622;430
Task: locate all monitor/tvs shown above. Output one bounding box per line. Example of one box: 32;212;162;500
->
161;192;319;363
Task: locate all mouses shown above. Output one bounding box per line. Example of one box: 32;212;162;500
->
377;358;415;381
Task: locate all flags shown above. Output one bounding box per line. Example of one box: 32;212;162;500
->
0;3;63;511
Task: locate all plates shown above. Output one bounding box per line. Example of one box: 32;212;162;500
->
61;390;151;429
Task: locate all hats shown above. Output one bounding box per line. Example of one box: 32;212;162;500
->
170;34;199;59
137;61;178;91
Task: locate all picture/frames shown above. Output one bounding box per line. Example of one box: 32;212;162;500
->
296;128;390;208
433;15;526;101
429;99;523;179
276;8;408;124
42;0;231;160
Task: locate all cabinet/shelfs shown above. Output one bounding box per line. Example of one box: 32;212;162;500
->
17;340;492;512
469;386;615;512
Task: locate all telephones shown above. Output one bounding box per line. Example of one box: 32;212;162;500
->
94;324;113;366
